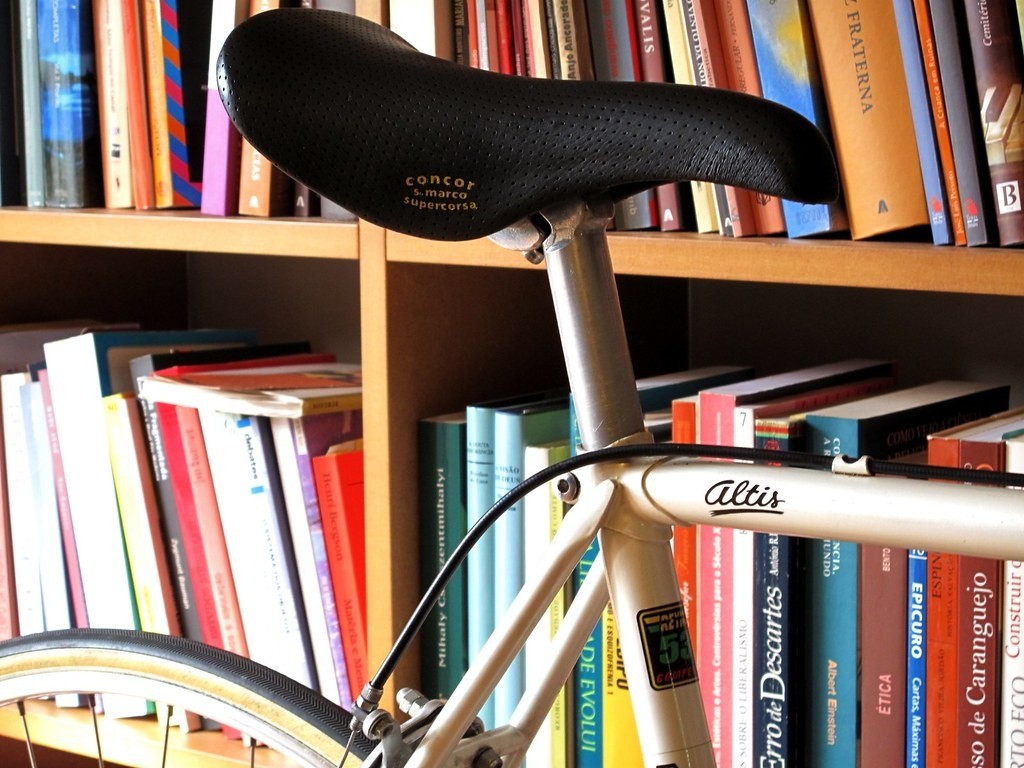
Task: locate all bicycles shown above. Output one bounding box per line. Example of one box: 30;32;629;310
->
1;9;1024;768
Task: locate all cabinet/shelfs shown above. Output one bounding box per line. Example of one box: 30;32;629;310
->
0;0;1023;768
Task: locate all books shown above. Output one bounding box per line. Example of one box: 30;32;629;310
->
0;0;1024;768
